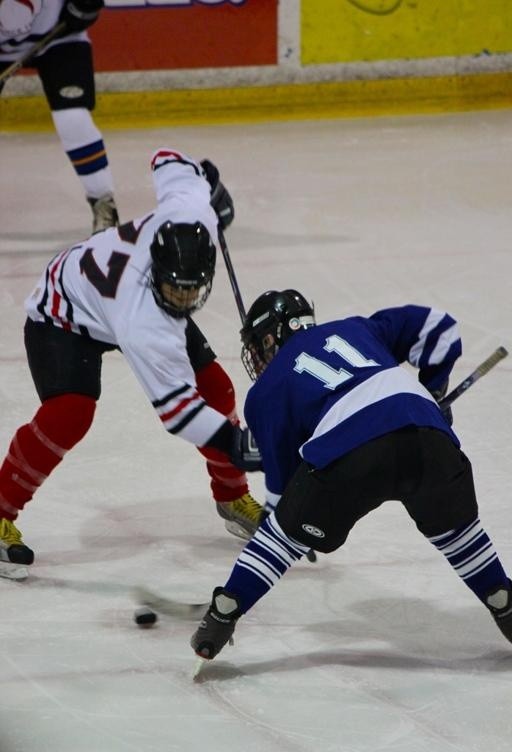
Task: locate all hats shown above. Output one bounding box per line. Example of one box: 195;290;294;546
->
241;287;317;381
149;218;217;319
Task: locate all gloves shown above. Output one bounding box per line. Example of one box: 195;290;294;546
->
200;158;234;230
58;0;104;35
229;427;263;472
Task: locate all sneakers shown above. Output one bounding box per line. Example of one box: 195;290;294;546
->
0;514;35;565
217;492;272;536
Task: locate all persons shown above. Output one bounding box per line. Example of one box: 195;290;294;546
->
2;148;268;570
1;2;120;237
188;290;511;663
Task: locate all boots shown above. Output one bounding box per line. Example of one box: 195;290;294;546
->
87;195;118;236
486;586;512;643
190;585;242;661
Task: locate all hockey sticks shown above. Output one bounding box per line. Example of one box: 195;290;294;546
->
134;347;506;622
